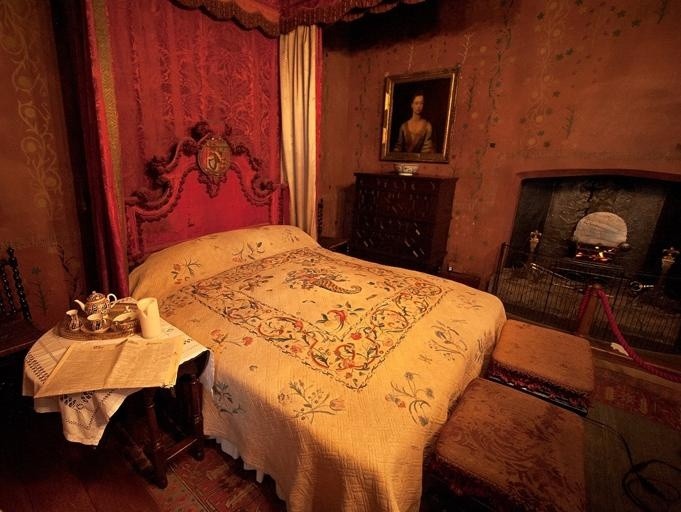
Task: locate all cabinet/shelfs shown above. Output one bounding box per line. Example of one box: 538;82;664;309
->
352;171;459;275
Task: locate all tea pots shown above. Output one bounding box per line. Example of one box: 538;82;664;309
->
74;291;117;316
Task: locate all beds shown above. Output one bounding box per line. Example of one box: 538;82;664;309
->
123;121;506;512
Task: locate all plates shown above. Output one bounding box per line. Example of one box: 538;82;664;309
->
81;318;112;335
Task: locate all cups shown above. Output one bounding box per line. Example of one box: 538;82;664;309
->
87;313;107;331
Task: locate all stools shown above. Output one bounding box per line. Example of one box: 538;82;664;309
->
430;319;595;512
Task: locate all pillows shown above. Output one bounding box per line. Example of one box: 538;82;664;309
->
128;224;316;305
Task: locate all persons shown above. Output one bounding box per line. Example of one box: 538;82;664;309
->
395;95;437;154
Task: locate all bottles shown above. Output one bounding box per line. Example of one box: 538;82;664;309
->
66;310;84;333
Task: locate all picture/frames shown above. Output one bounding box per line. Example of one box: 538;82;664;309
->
379;63;460;164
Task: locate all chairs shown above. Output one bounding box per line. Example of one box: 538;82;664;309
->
0;245;41;358
318;198;350;255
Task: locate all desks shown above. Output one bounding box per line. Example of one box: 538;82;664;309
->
21;297;215;489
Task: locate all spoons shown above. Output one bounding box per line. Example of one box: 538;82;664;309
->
113;317;138;327
